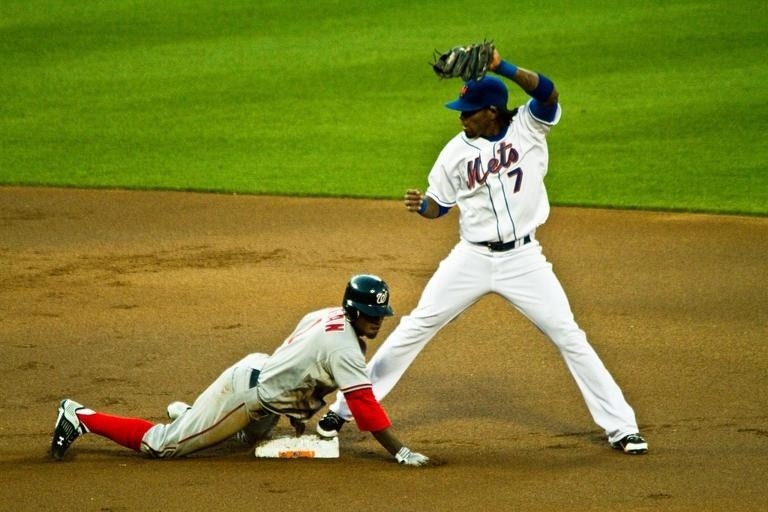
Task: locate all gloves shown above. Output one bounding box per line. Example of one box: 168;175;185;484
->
395;445;432;468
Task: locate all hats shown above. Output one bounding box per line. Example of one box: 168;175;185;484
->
443;76;509;114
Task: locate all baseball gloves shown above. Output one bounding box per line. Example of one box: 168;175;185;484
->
432;37;495;83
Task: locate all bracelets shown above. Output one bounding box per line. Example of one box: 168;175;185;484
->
420;199;427;213
496;59;518;78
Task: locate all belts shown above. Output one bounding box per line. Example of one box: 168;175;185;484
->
249;368;269;417
477;233;531;252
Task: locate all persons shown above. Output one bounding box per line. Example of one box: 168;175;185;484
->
50;273;431;467
316;45;649;454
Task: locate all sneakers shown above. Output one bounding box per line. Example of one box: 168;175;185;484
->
617;433;650;455
166;400;193;419
316;409;346;438
50;398;85;461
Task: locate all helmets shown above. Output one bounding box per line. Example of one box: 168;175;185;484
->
342;273;395;318
